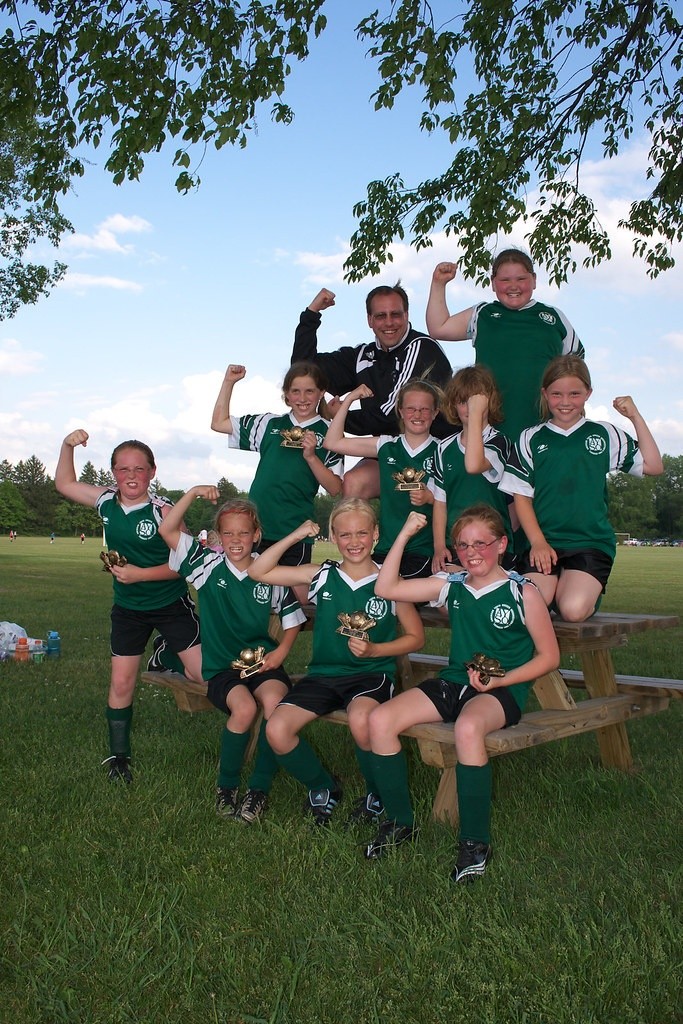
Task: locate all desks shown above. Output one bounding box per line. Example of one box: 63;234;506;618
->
302;613;683;828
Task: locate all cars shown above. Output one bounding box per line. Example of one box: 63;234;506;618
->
623;538;683;548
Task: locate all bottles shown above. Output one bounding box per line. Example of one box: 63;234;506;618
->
15;639;30;662
32;640;44;664
47;630;61;661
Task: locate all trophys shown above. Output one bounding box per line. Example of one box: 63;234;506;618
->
464;652;506;686
280;426;306;449
231;646;266;679
99;550;127;572
335;611;376;642
392;468;426;491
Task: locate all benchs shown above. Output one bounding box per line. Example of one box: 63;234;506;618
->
142;653;683;756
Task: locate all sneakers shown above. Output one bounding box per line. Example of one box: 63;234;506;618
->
239;789;269;822
343;792;386;835
364;819;418;860
101;754;132;786
308;789;342;828
216;784;238;815
449;838;493;886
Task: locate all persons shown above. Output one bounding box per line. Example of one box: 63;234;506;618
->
198;528;223;553
9;529;17;543
80;533;85;545
50;532;54;543
158;486;307;823
55;430;203;784
364;504;560;886
247;497;425;834
211;248;663;623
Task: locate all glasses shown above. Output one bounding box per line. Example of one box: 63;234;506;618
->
402;408;434;414
371;312;402;320
457;538;499;550
